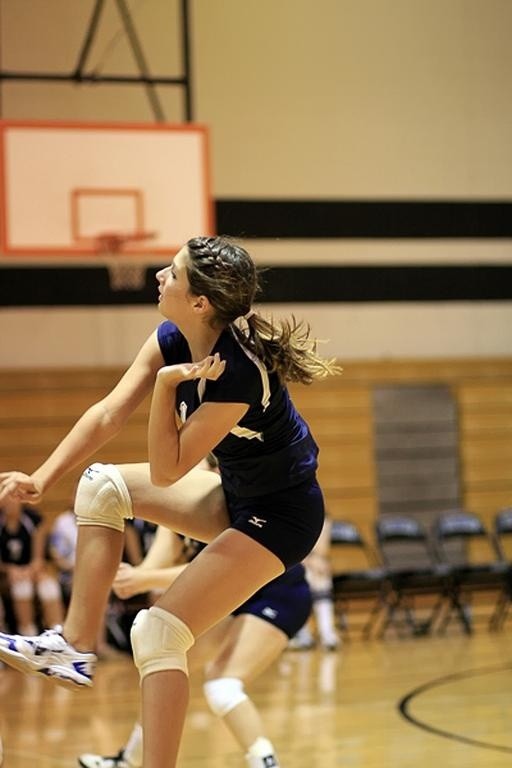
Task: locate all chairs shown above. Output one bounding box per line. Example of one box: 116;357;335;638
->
330;509;512;642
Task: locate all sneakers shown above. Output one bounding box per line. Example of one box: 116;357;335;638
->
0;625;97;688
79;754;135;768
296;633;339;648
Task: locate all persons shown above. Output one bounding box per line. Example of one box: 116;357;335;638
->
2;453;341;660
1;236;326;767
79;450;314;768
